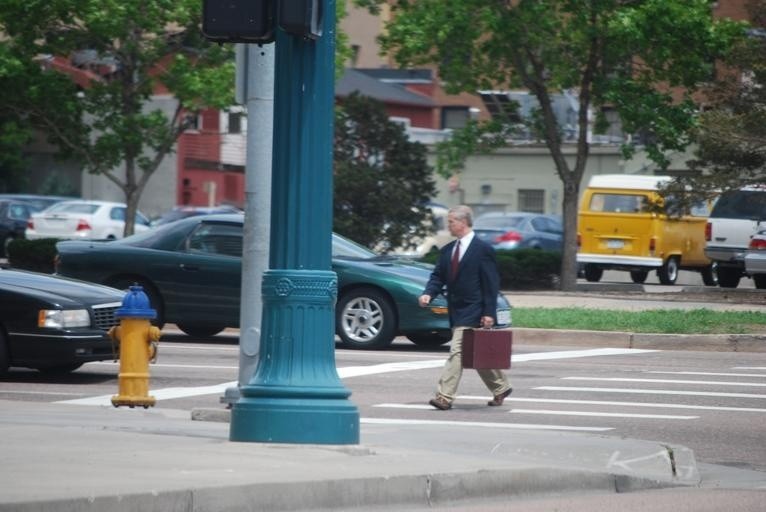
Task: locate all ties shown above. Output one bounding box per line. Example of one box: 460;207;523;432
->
451;241;460;281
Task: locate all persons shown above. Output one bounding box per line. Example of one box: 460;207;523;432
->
418;205;512;410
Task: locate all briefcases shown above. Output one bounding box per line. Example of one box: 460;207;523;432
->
462;328;513;369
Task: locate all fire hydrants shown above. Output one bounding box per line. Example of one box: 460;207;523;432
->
107;282;161;409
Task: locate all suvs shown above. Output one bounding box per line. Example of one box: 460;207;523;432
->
577;175;719;287
705;183;766;292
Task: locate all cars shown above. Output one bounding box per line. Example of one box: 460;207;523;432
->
26;200;152;260
1;262;133;377
149;206;241;230
52;214;514;353
469;212;585;278
375;207;457;257
0;194;80;267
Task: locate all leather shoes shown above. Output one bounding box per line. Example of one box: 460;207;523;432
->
488;386;512;406
429;395;453;410
